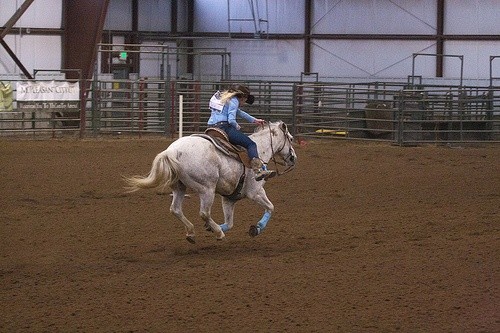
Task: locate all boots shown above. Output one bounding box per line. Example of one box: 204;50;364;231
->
250;157;276;181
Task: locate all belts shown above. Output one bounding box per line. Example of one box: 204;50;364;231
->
209;121;228;128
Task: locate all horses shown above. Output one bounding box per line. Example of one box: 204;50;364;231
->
119;120;298;247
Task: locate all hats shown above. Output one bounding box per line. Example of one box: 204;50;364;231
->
231;85;254;105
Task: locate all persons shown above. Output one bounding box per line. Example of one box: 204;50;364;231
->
206;84;276;181
